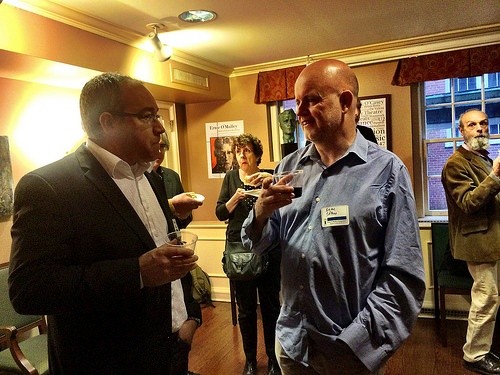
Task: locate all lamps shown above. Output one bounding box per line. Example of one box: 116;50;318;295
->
146;23;173;62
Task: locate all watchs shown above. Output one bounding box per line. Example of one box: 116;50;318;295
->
186;316;202;328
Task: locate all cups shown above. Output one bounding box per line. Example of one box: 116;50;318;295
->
275;171;303;198
164;231;198;257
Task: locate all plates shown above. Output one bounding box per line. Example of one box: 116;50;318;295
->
172;193;205;202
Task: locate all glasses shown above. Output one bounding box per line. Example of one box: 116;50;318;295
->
235;149;254;155
109;110;162;123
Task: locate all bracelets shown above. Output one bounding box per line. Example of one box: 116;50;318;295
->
167;199;175;212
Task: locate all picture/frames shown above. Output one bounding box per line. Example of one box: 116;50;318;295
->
357;93;393;152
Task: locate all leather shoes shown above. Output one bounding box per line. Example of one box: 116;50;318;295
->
463;351;500;375
242;358;257;375
266;359;281;375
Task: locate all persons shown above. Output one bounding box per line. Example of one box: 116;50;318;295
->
215;59;427;375
212;137;239;174
277;108;296;143
441;107;500;374
7;74;203;375
147;129;203;231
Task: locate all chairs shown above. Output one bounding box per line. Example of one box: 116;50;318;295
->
431;223;474;347
0;262;49;375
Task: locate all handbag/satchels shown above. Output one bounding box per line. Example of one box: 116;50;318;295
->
187;263;214;306
221;241;264;279
